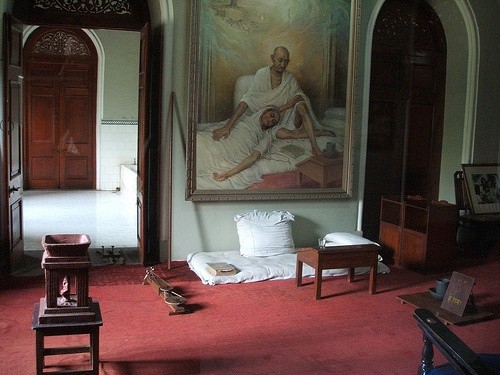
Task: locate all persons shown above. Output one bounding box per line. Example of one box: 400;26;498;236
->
474;178;496;198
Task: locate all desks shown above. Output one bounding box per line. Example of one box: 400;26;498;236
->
295;243;380;300
459;212;500;265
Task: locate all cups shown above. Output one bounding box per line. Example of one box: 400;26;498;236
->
435;278;449;295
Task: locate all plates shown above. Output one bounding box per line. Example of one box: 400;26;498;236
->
429;288;444;301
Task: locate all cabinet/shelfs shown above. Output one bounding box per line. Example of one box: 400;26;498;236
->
379;193;461;274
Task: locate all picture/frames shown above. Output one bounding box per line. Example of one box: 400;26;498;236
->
185;0;362;204
461;163;500;216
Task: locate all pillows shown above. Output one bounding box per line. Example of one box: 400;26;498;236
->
324;232;381;249
317;238;383;272
232;209;296;258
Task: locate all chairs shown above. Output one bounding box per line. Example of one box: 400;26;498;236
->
412;308;500;375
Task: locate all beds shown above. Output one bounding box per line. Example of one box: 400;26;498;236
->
187;245;391;287
